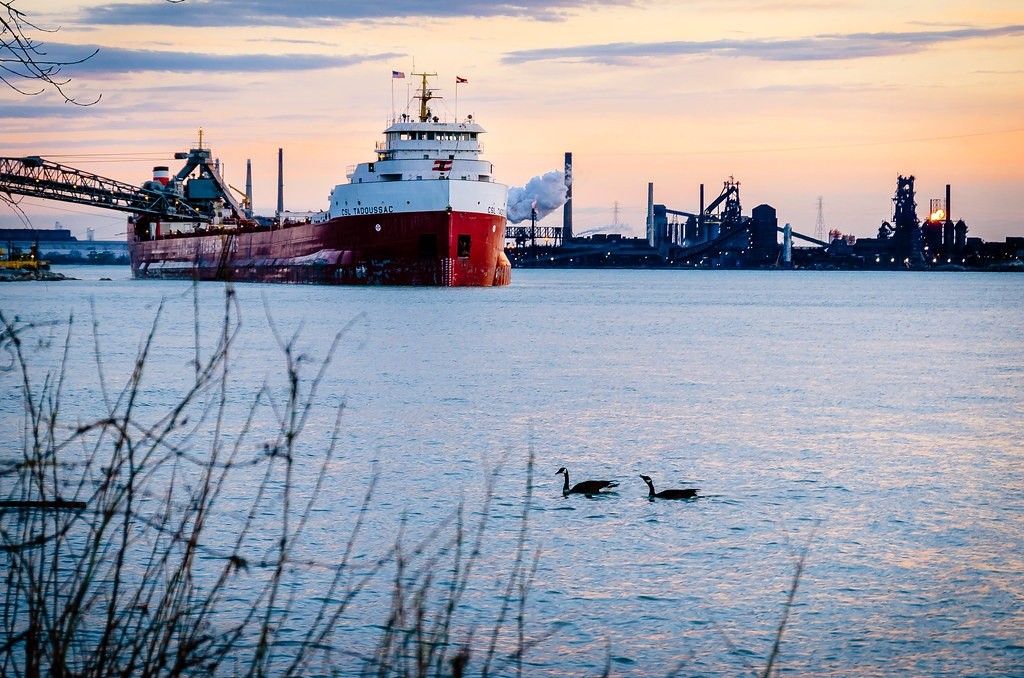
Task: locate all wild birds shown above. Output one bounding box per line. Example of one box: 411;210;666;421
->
639;473;701;500
555;467;614;495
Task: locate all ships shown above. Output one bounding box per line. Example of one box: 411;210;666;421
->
124;64;513;290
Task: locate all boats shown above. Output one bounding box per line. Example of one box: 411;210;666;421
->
0;235;66;281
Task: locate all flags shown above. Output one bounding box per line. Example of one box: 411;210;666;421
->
393;70;405;78
456;75;468;83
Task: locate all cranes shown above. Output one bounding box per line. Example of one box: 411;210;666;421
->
0;149;210;223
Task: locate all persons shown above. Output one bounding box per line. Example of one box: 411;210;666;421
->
426;109;432;122
